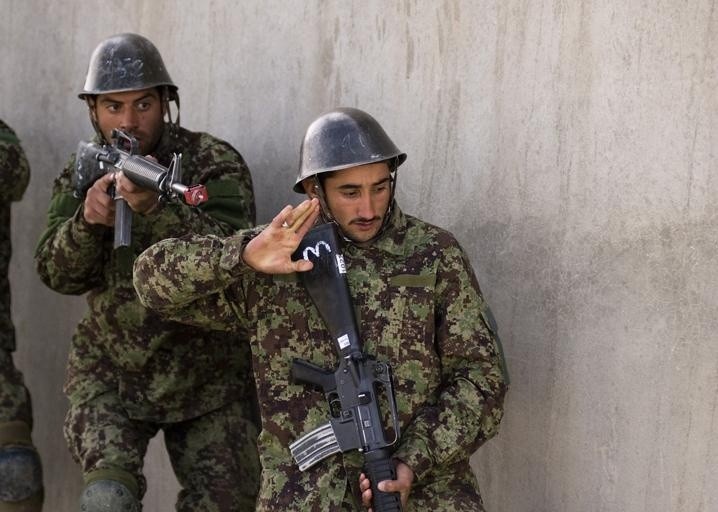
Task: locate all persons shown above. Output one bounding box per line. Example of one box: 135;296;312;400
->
0;116;42;512
133;105;511;512
32;31;263;512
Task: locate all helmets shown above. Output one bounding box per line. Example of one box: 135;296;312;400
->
77;33;178;100
293;107;407;194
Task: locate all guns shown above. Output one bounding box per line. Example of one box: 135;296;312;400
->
288;222;404;512
73;128;209;250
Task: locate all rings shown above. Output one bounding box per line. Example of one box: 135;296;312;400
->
282;221;290;230
